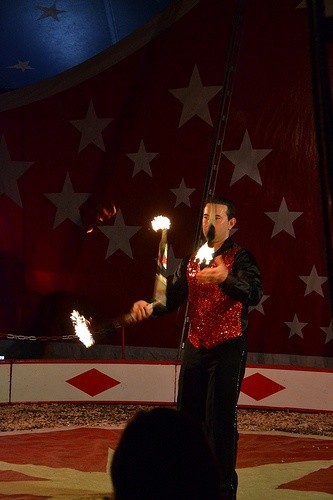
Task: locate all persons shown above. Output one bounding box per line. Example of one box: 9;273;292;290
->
130;188;264;499
111;403;228;500
